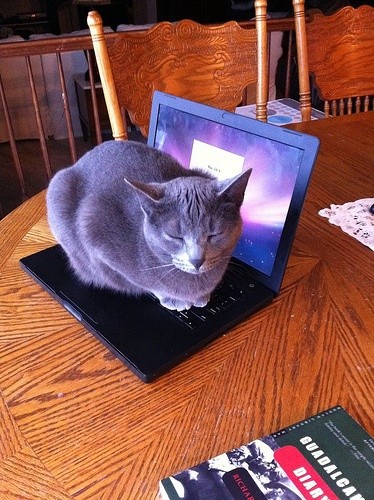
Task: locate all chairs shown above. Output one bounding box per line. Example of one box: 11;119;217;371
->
87;0;374;142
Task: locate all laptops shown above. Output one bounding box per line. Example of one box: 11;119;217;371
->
20;90;319;383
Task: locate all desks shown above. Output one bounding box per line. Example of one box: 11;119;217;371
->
0;111;374;500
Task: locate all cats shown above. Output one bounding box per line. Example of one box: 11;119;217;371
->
46;141;253;312
